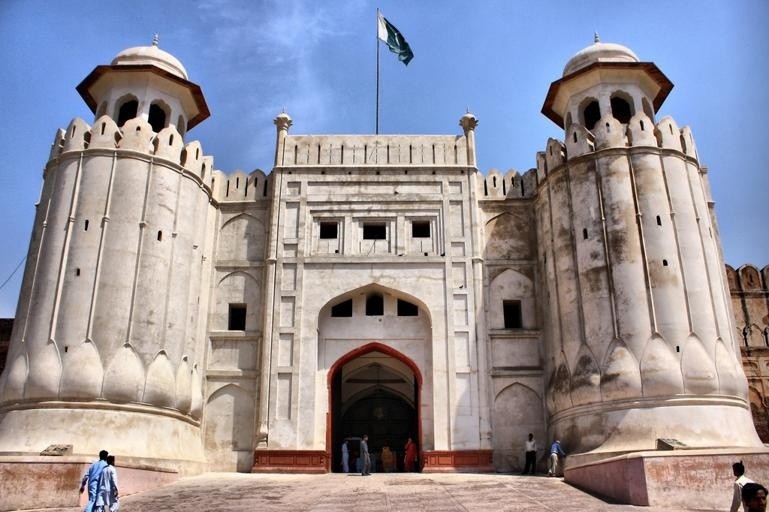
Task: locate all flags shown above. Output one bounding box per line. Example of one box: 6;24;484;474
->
377;8;414;67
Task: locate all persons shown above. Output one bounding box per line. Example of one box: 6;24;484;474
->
742;482;768;512
545;440;565;477
360;434;371;476
403;437;418;472
341;440;350;473
521;433;537;475
729;463;754;512
80;450;109;512
95;455;120;512
